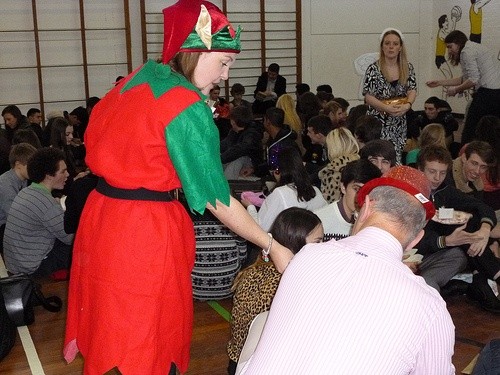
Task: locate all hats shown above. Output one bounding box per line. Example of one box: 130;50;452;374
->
355;164;437;220
154;0;242;80
380;27;404;42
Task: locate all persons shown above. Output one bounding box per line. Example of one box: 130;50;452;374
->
452;141;500;229
407;145;500;314
234;165;456;375
361;27;417;166
425;30;500;142
254;63;287;113
2;147;73;312
1;144;39;253
314;160;465;302
63;0;294;375
225;207;323;375
0;82;458;187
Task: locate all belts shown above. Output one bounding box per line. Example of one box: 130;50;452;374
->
96;176;176;202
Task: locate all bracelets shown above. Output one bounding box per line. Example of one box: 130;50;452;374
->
261;233;273;262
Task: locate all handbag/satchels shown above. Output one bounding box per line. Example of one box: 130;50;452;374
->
378;98;409;110
0;273;63;326
